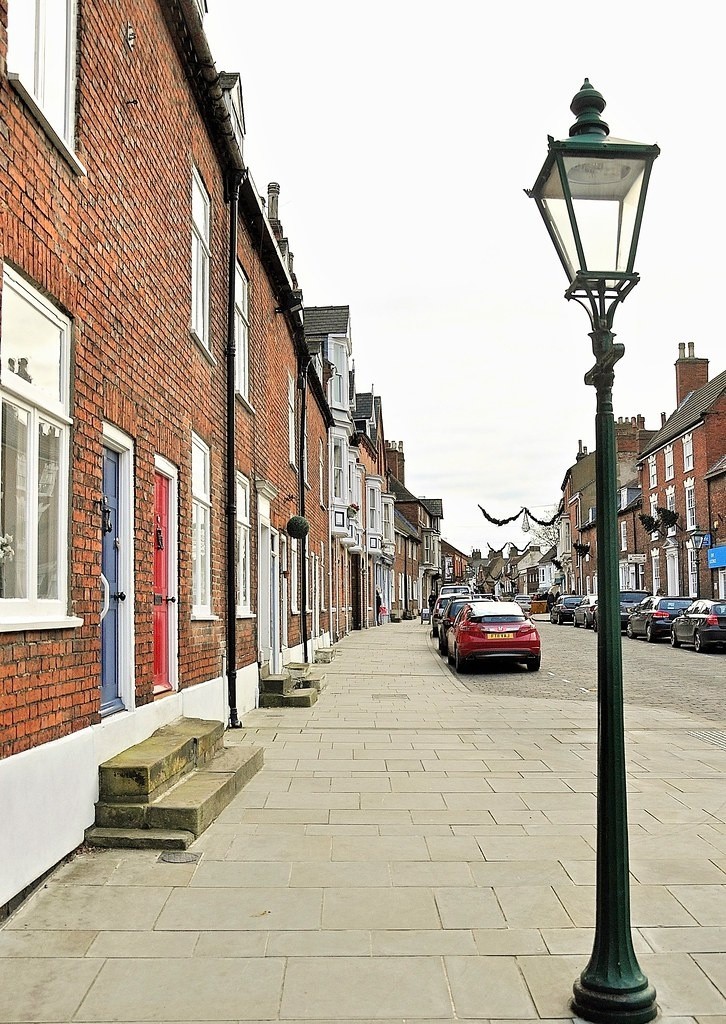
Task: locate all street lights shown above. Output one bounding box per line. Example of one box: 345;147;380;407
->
689;522;706;598
525;78;661;1022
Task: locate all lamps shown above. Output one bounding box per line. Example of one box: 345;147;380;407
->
635;461;646;471
271;288;303;315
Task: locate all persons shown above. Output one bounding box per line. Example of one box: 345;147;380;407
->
376;590;382;626
471;587;487;598
644;586;653;597
656;588;665;596
531;591;561;611
572;590;576;594
491;588;495;601
428;591;436;613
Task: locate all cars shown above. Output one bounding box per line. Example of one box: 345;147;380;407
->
427;583;541;677
514;586;551;610
550;589;726;652
